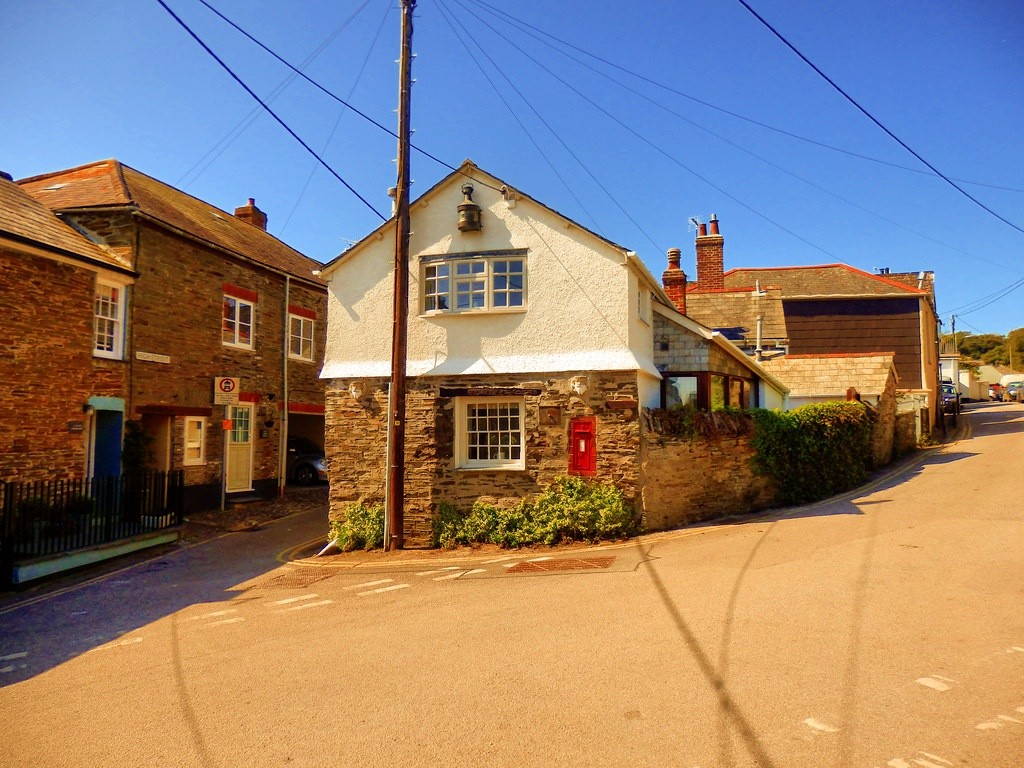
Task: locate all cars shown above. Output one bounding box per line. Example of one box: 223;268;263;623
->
286;435;329;488
939;375;963;416
986;381;1024;403
